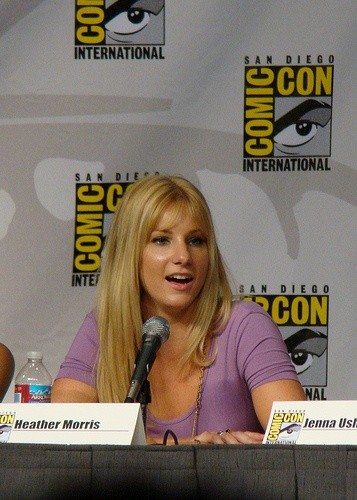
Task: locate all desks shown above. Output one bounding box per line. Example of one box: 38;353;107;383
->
0;442;357;500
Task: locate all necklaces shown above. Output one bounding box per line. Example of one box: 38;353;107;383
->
191;334;207;437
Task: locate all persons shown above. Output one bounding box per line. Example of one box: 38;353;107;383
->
51;175;308;444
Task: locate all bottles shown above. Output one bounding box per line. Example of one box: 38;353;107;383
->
15;351;53;403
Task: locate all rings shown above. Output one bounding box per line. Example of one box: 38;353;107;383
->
217;431;224;435
226;429;231;433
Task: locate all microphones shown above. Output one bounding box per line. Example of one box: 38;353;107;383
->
124;315;170;404
